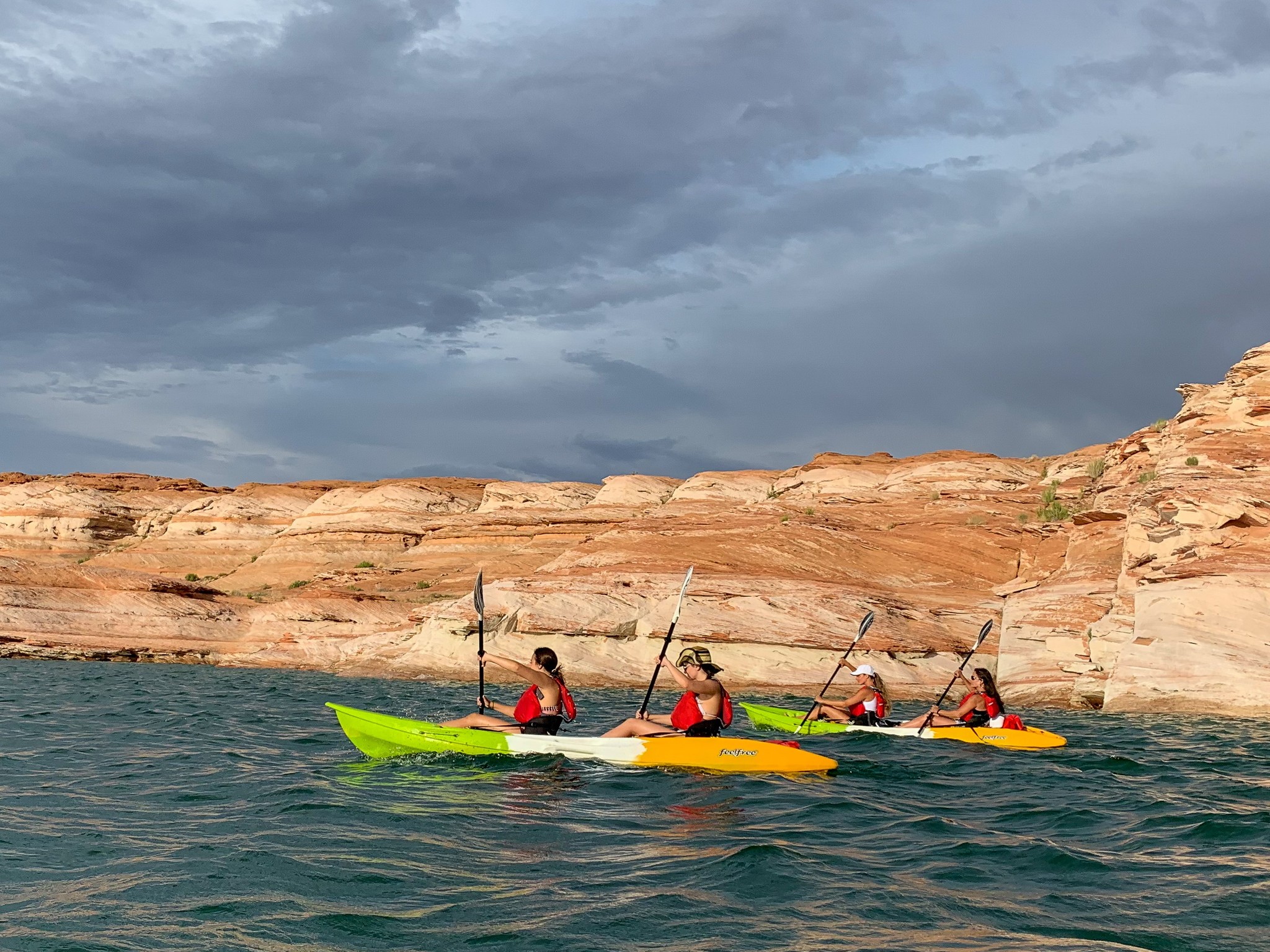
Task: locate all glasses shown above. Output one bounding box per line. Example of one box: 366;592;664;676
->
971;676;982;681
684;663;692;671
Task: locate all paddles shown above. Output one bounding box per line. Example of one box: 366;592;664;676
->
913;620;994;740
795;611;876;735
638;562;697;722
473;569;486;725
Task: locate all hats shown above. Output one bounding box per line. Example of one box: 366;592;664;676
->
676;646;724;674
850;665;875;676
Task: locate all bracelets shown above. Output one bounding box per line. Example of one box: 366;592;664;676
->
937;708;942;715
492;701;494;710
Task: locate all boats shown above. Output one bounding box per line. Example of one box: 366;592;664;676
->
325;701;839;777
738;701;1067;752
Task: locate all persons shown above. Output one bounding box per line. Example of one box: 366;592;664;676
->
894;668;1005;727
599;646;724;737
785;657;884;726
438;647;566;736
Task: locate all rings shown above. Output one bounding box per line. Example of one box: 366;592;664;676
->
814;699;815;700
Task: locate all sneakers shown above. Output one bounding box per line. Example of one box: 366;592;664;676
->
875;722;894;727
885;719;901;726
